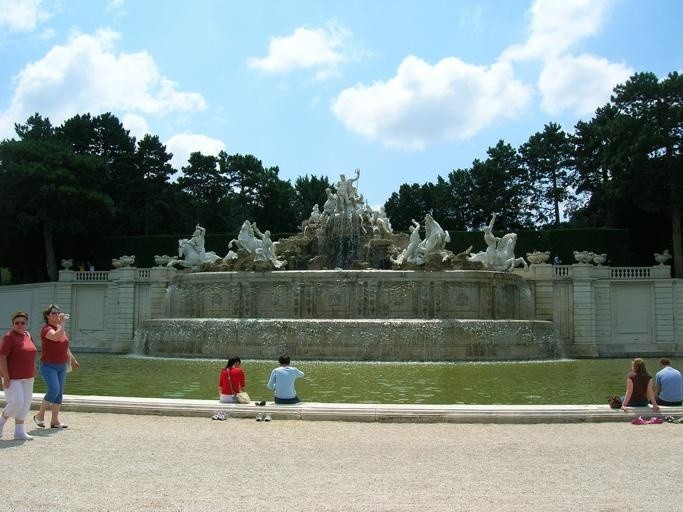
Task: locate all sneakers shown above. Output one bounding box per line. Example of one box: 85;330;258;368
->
212;412;229;420
14;433;34;440
256;413;272;421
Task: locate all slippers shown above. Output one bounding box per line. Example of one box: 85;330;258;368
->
51;423;68;428
34;415;45;427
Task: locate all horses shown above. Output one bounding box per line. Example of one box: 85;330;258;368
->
166;239;223;268
420;214;451;252
227;220;263;257
469;232;529;272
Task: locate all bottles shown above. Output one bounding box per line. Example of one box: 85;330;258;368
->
62;313;69;321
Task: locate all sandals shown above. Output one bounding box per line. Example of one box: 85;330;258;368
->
632;416;683;425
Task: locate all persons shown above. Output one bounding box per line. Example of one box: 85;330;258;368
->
405;218;422;258
187;225;206;254
265;354;305;404
217;356;245;403
250;222;273;259
306;169;390;233
652;359;682;406
619;358;660;411
480;212;505;268
0;312;37;440
32;303;78;429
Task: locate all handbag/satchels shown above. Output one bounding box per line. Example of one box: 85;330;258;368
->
236;392;250;404
608;396;622;408
